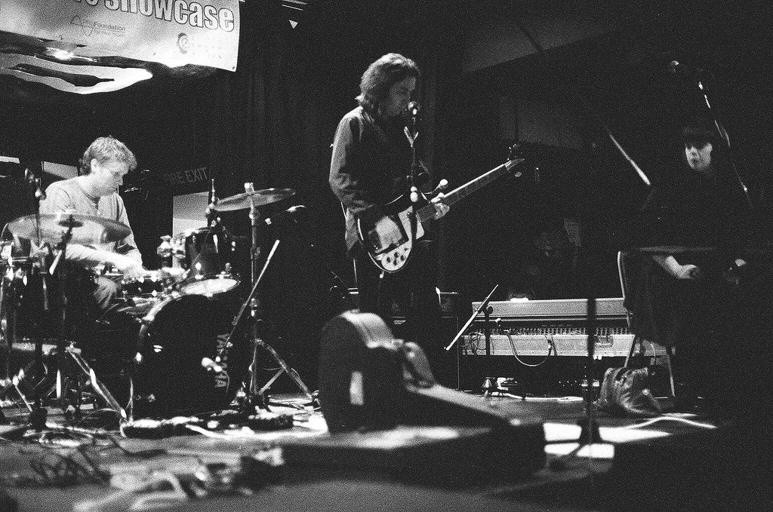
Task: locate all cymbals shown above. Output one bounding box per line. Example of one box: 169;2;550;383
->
208;188;296;212
8;214;130;244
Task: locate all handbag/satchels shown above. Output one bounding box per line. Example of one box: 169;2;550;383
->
593;366;663;419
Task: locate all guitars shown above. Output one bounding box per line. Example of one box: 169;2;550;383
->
354;144;526;273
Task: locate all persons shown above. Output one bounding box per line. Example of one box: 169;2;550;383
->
641;119;750;383
36;134;145;347
327;48;456;386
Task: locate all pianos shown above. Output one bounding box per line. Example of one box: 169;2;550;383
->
461;299;675;357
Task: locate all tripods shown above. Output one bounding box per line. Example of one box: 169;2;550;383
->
441;283;518;401
0;234;128;433
230;217;317;417
542;164;619;468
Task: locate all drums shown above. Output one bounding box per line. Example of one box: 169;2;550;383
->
123;296;222;387
119;271;174;314
172;226;242;295
0;255;102;345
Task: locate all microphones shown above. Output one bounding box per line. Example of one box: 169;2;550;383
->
406;101;422;115
669;59;680;74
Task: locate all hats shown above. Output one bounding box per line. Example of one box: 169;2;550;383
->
677;124;715;149
